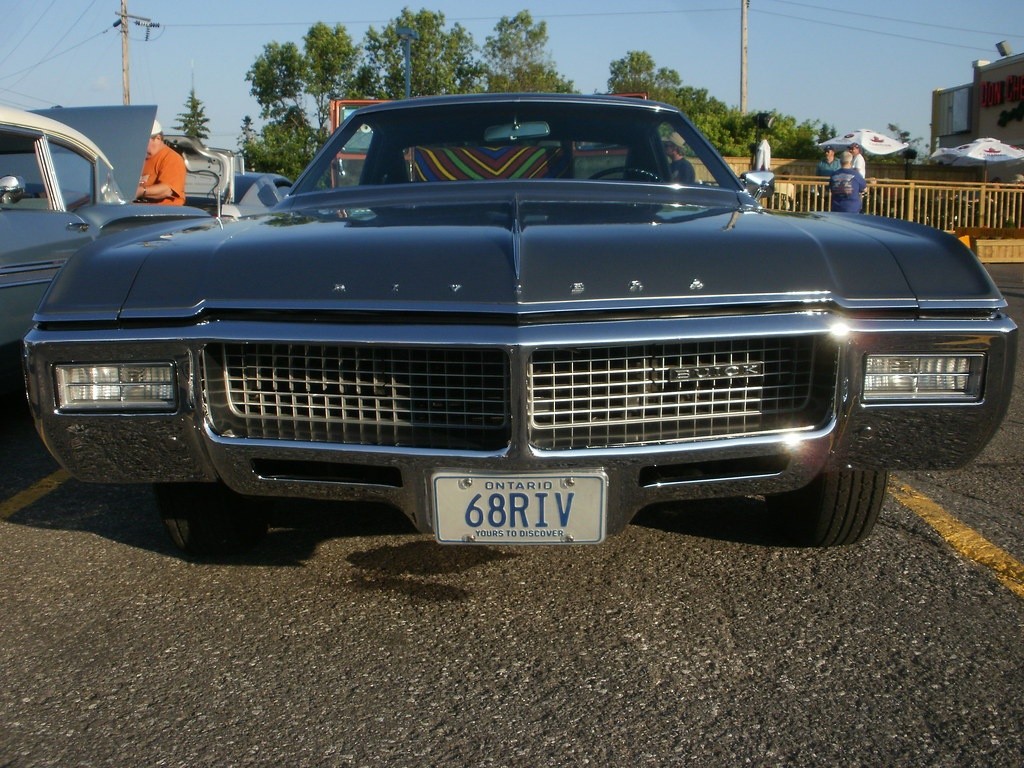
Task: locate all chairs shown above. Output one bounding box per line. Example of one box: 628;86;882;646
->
768;189;822;211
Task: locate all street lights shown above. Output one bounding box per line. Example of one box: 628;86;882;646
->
397;27;419;99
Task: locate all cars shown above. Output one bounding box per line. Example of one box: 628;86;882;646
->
0;105;214;423
161;135;293;225
20;91;1020;559
185;170;294;220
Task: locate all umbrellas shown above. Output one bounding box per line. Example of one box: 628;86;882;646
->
755;140;771;172
818;129;910;156
929;137;1024;182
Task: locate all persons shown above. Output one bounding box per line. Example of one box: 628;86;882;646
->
135;119;187;206
770;172;790;209
816;145;841;211
661;132;696;188
829;152;867;214
847;143;865;180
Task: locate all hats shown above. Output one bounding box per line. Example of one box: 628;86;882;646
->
150;119;162;136
847;142;860;149
823;145;835;152
661;132;686;148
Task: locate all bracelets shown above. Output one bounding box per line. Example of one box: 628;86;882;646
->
143;187;146;196
